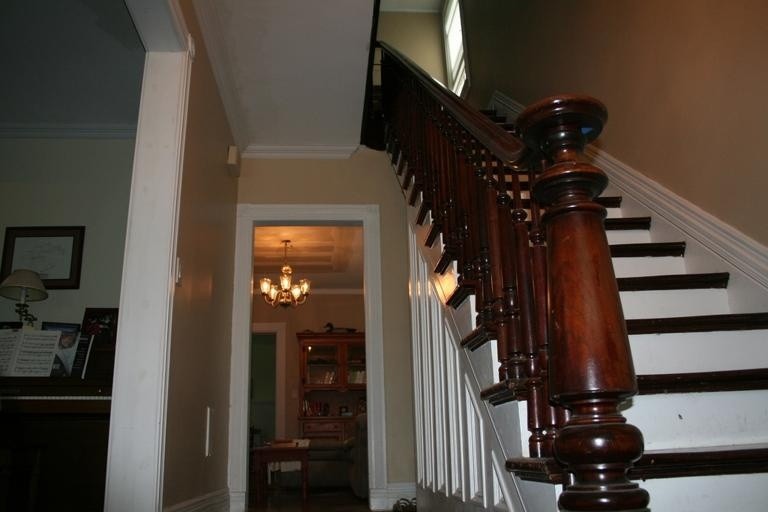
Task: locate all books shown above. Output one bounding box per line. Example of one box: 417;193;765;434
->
302;348;366;417
40;321;85;378
268;441;298;448
79;306;120;386
0;327;60;380
70;333;97;383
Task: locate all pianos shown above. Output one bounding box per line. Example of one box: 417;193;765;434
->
0;389;111;414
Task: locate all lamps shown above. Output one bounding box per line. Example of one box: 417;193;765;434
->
0;267;51;322
257;238;311;313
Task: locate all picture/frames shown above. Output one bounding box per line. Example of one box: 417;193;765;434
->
0;221;86;291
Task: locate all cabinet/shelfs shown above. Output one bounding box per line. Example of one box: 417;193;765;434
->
293;331;364;500
